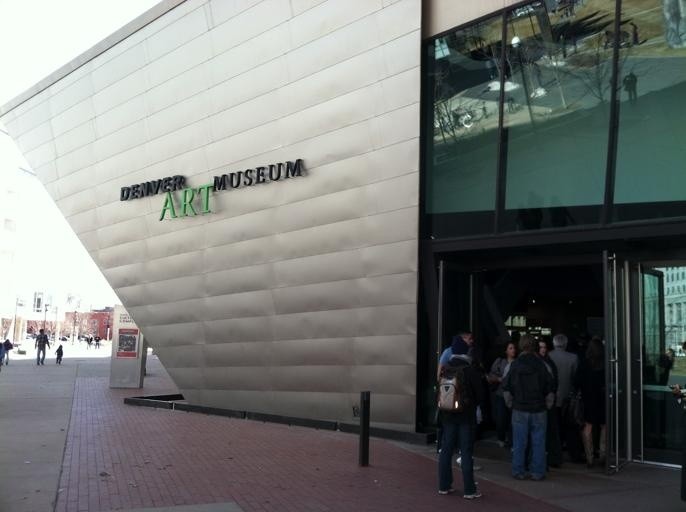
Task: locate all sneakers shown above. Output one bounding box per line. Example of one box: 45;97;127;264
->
463;492;482;499
438;488;456;494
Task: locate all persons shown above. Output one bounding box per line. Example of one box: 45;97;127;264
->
622;67;640;106
0;339;13;371
656;348;675;385
437;330;607;499
55;345;63;365
86;333;101;350
35;328;51;365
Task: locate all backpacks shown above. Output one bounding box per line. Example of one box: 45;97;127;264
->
437;362;476;412
